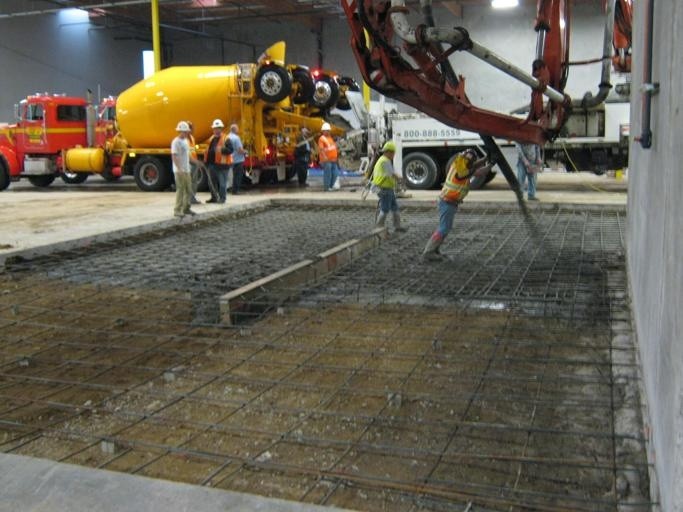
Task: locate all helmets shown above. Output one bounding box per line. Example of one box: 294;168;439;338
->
175;121;191;133
321;123;331;130
464;148;478;161
211;119;224;128
382;141;395;152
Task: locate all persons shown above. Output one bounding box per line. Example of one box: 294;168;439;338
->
319;121;340;192
294;127;311;187
171;120;204;217
204;119;233;204
515;141;542;200
227;124;248;196
185;120;200;204
421;148;490;261
372;141;407;234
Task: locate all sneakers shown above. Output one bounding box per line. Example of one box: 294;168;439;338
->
395;226;406;233
528;198;539;200
426;252;442;261
174;208;195;217
206;198;225;203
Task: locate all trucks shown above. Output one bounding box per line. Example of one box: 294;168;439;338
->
330;90;629;190
